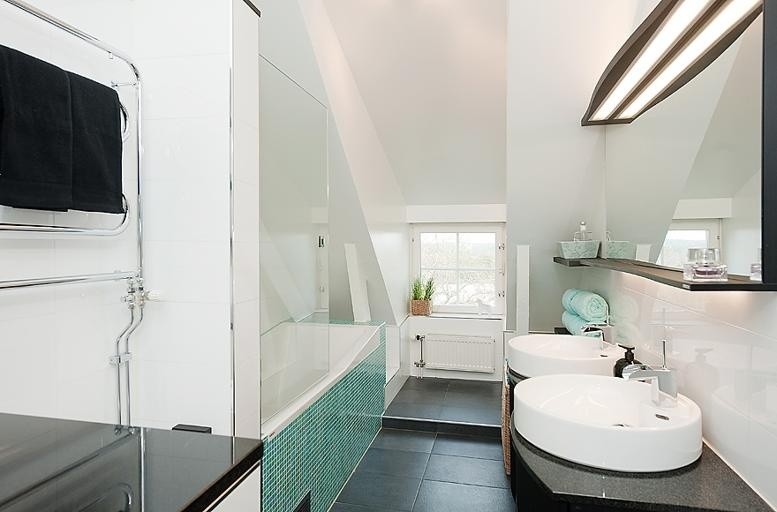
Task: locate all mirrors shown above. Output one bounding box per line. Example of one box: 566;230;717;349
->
607;13;763;280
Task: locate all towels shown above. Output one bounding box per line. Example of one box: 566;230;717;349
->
561;288;609;336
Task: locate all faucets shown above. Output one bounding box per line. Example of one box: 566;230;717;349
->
582;305;618;346
615;340;679;404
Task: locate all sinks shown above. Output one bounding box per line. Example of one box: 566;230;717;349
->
514;376;702;474
508;332;628;377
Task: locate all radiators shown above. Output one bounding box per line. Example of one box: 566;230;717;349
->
413;333;496;378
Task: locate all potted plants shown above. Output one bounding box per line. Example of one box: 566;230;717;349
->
410;277;435;315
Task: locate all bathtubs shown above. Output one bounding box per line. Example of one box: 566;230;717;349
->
261;320;381;443
